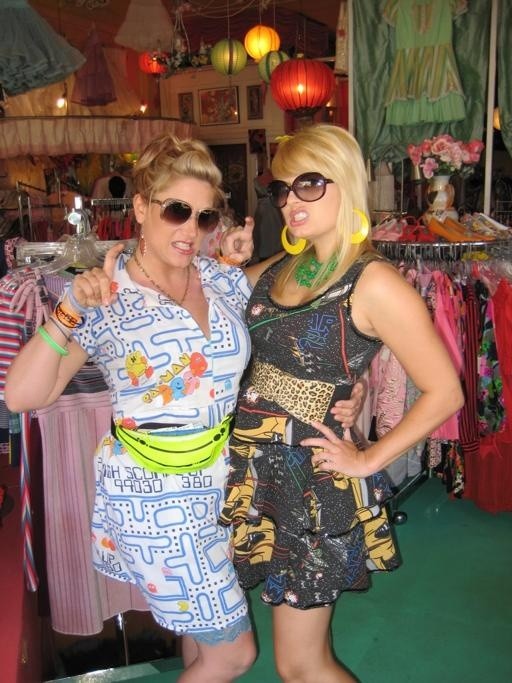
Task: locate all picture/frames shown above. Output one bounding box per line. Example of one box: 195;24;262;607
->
197;85;240;126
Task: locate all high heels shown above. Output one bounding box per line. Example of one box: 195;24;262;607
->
371;212;512;244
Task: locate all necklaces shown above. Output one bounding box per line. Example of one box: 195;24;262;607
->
129;250;190;305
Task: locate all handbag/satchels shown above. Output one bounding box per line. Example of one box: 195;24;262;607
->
116;415;230;473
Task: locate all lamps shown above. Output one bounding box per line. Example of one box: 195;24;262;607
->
210;0;339;127
137;38;170;85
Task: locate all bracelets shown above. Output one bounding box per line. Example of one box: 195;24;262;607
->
38;325;71;356
48;284;100;344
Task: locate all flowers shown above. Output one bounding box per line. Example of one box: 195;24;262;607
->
407;134;483;181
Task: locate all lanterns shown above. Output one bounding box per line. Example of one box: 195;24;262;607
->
269;55;337;121
139;50;170;83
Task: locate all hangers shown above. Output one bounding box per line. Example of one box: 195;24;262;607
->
34;205;105;280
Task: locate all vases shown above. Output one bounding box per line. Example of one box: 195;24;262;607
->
420;175;459;224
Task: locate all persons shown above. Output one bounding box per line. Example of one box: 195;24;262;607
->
3;130;368;682
217;120;469;683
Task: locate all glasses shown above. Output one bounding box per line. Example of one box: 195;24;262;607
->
267;172;335;208
145;199;221;234
